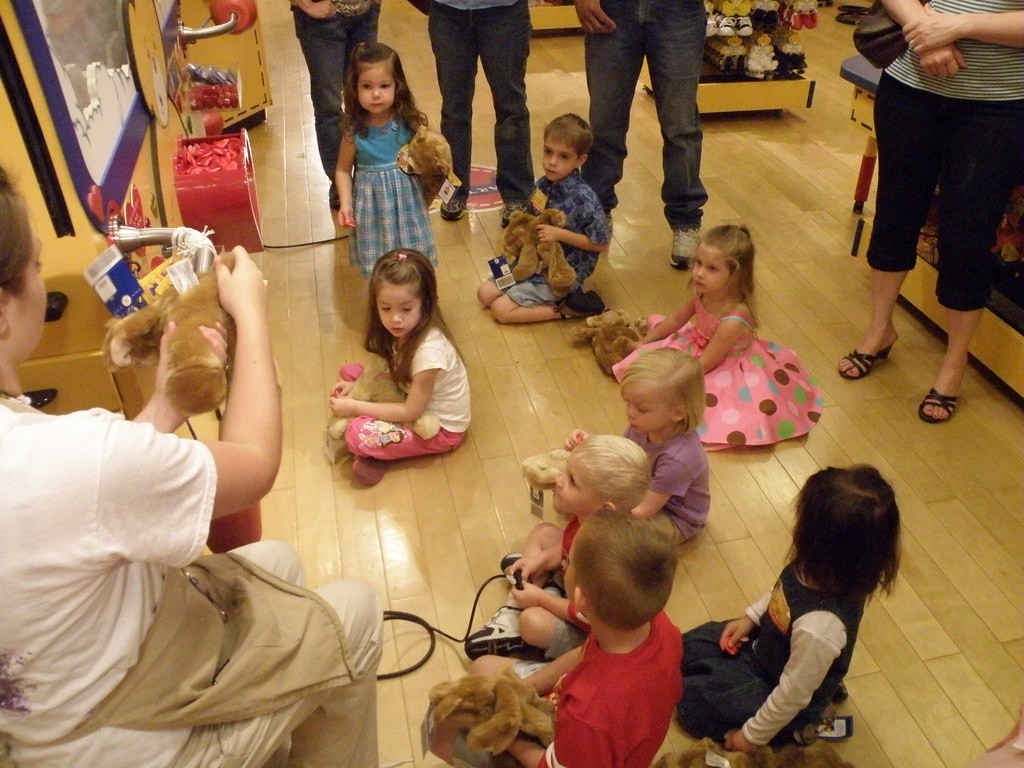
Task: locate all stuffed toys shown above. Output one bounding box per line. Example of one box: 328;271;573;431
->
101;252;284;414
569;308;650;374
655;737;853;768
398;126;453;208
503;209;577;297
522;449;571;488
429;665;554;756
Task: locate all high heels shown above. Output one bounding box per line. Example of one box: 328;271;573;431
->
919;386;958;423
837;334;899;380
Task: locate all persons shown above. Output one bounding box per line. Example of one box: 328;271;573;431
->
329;248;472;489
0;162;382;768
612;224;824;452
838;0;1024;424
574;0;708;265
477;112;605;323
337;43;439;282
469;346;900;768
406;0;536;229
290;0;382;211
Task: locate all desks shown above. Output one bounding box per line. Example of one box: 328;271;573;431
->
840;56;885;214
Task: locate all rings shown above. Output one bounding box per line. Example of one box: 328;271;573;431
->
909;40;916;48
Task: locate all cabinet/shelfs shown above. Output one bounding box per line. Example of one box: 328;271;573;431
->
178;0;273;135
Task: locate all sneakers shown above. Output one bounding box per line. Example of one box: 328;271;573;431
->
704;13;753;37
439;197;472;220
670;225;702;268
499;199;531;228
465;606;541;660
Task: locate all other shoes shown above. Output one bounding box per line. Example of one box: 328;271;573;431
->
792;699;837;746
329;178;341;208
352;452;389;485
553;288;605;319
340;361;388;386
833;679;849;701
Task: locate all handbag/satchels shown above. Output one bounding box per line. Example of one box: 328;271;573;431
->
852;0;931;69
